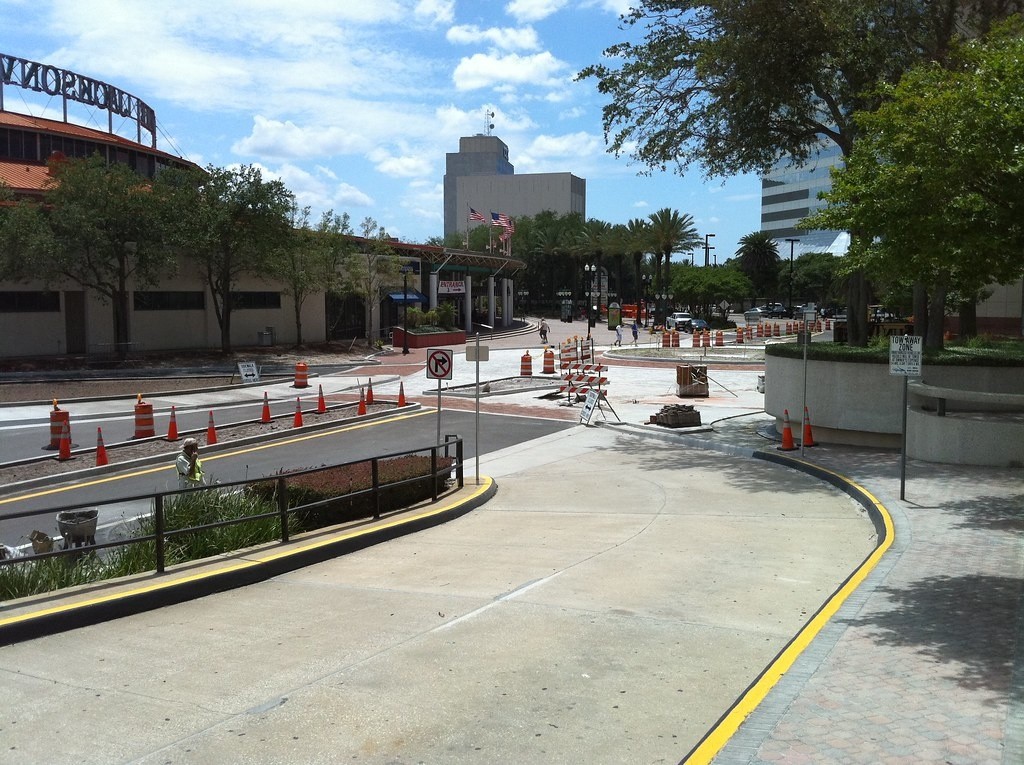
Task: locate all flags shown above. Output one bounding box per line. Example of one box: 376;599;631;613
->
498;221;517;242
468;206;486;223
490;212;508;227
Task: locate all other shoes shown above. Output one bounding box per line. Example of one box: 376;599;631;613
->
630;342;639;347
614;342;616;347
618;344;621;347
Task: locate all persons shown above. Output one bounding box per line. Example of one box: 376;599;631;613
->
630;320;641;346
614;324;624;347
539;318;551;342
176;437;209;497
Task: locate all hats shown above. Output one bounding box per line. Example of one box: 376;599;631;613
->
179;438;198;448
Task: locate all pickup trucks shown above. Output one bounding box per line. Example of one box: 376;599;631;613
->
666;313;692;330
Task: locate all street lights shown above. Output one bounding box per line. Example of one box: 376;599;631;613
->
702;234;717;266
585;264;597;339
784;239;799;317
642;274;653;327
688;253;693;267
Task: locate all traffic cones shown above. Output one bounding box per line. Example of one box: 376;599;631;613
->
53;420;75;461
314;384;330;413
398;382;406;407
358;388;366;415
797;407;818;447
294;397;303;428
365;378;375;405
257;392;275;423
96;427;108;465
777;410;799;450
162;406;183;441
207;411;217;445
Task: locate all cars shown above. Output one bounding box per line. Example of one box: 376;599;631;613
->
684;319;710;334
743;303;819;322
